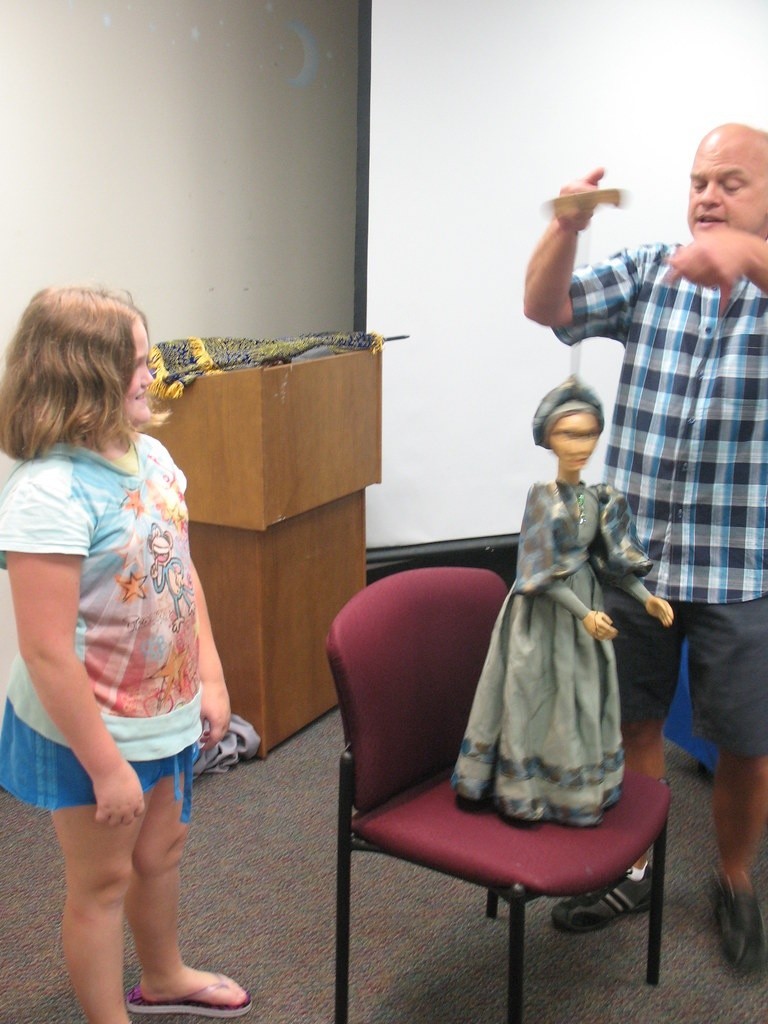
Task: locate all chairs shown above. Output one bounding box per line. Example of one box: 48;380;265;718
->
327;568;671;1024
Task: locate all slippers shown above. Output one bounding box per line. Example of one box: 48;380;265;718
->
126;972;252;1018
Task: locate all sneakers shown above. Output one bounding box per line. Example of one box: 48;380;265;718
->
714;880;764;965
551;862;651;931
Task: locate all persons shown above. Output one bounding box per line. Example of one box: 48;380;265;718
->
523;123;766;982
1;287;252;1024
451;381;674;829
